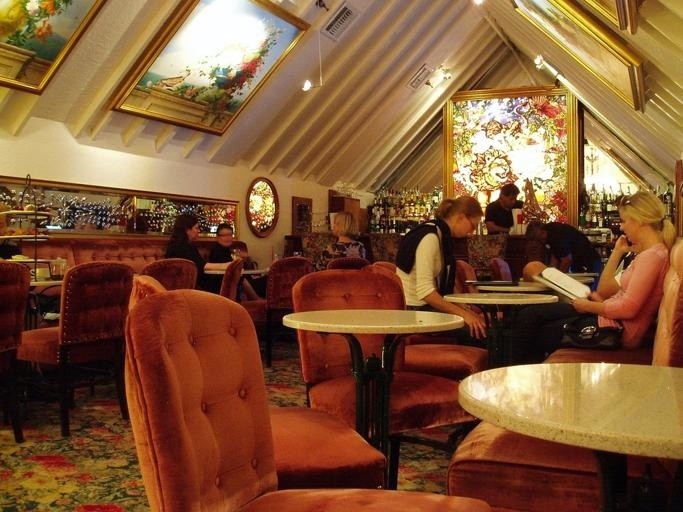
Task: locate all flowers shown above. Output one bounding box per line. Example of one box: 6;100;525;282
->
532;98;565;140
198;15;284;91
20;0;70;43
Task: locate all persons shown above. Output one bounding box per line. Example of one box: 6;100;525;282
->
591;234;630;300
208;222;266;298
312;211;367;272
165;214;260;300
485;183;520;236
526;220;604;293
516;192;678;362
396;195;518;368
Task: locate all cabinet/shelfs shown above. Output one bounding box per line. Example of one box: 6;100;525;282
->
584;199;675;243
330;195;360;231
370;195;439;234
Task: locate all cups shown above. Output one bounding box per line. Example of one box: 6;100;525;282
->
29;267;51;281
231;247;241;261
51;256;68;280
293;251;303;257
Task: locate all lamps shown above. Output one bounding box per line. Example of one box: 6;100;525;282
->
533;56;545;71
424;61;455;92
296;1;326;93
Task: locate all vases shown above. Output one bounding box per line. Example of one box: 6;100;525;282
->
1;0;20;37
211;72;237;91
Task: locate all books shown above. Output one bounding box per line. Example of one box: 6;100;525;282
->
535;267;591;301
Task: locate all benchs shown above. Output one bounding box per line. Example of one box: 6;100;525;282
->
19;238;250;266
446;237;682;510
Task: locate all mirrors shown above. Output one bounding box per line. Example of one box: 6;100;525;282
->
245;177;280;239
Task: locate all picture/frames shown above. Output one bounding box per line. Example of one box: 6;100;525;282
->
583;0;639;35
440;83;581;232
509;0;647;116
0;0;107;97
105;1;313;140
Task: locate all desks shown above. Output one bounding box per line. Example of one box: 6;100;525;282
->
446;273;595;356
459;363;683;511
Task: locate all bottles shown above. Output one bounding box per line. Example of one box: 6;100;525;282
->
579;181;675;271
369;183;443;234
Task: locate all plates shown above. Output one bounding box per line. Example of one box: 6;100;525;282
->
4;258;50;266
0;234;50;243
0;210;54;220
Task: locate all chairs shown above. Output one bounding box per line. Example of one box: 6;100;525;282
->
3;257;491;510
